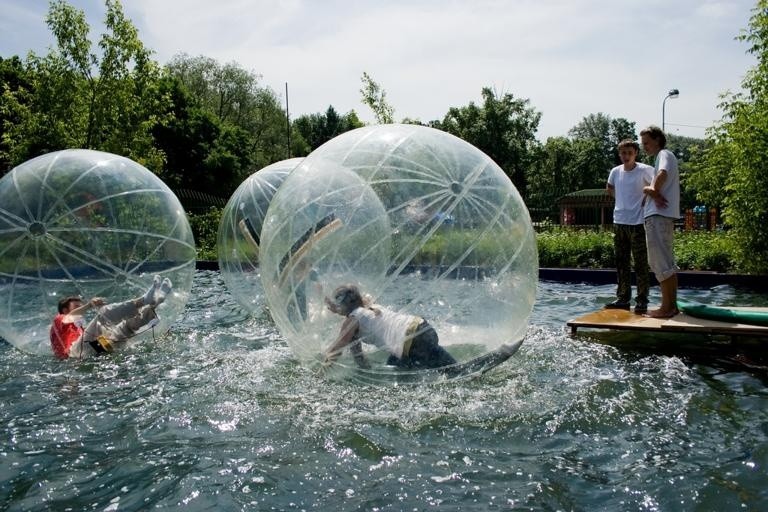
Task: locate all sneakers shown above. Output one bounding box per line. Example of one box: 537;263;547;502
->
635;302;647;315
604;298;630;310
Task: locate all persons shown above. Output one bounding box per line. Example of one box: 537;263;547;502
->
641;126;681;318
605;139;655;315
324;284;469;383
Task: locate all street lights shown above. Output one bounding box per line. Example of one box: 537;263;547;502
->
663;89;679;133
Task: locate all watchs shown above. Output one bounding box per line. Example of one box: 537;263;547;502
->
50;275;173;360
